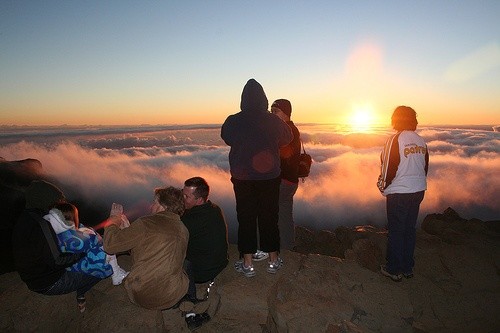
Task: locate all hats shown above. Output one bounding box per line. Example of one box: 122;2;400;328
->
271;99;292;117
25;179;65;209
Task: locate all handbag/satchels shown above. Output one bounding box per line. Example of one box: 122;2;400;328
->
298;153;312;183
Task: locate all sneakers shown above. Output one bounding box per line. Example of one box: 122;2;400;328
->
379;264;415;282
252;250;269;260
265;256;284;273
181;295;212;330
234;260;256;277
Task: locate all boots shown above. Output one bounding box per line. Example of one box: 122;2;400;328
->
105;254;130;286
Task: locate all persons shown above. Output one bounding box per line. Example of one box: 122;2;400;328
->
221;79;294;278
103;185;211;330
48;202;128;286
11;179;113;296
180;177;229;284
252;99;301;262
377;106;429;282
